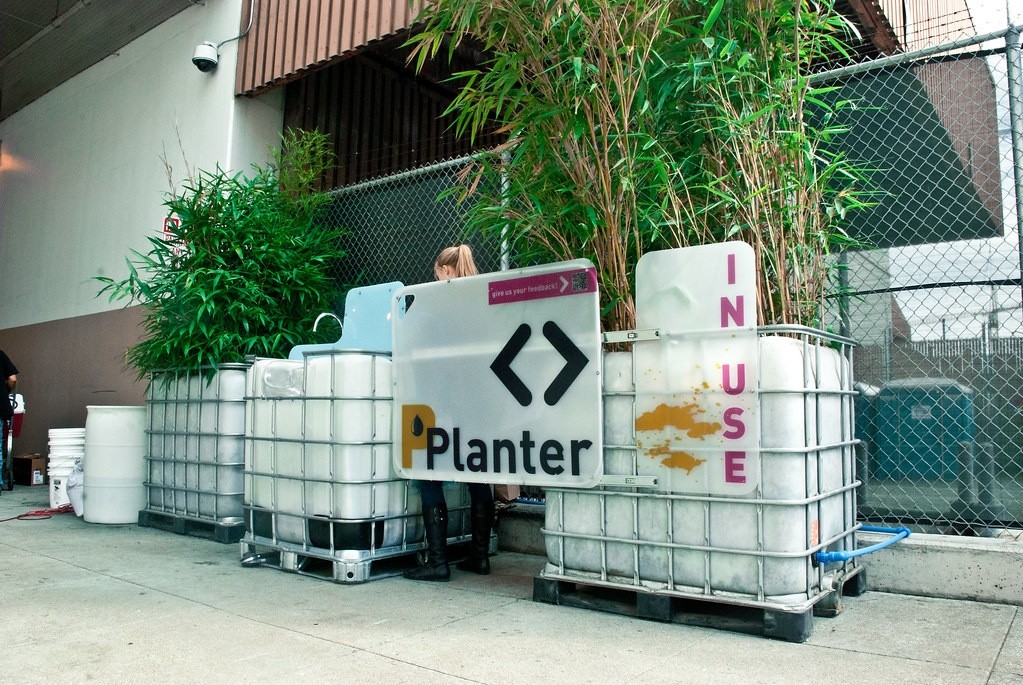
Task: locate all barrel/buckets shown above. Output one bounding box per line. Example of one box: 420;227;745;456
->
83;405;148;525
47;428;86;508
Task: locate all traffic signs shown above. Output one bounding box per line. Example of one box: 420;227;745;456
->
394;257;602;489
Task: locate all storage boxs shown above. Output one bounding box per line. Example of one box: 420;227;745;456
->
854;377;974;481
12;457;45;486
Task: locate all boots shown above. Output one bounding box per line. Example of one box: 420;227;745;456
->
404;504;451;581
457;499;493;575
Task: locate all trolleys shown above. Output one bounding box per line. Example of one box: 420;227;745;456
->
0;379;18;491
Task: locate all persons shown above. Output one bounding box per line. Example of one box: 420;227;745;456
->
403;244;494;581
0;349;19;495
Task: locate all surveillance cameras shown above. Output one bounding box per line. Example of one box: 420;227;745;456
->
191;43;219;73
